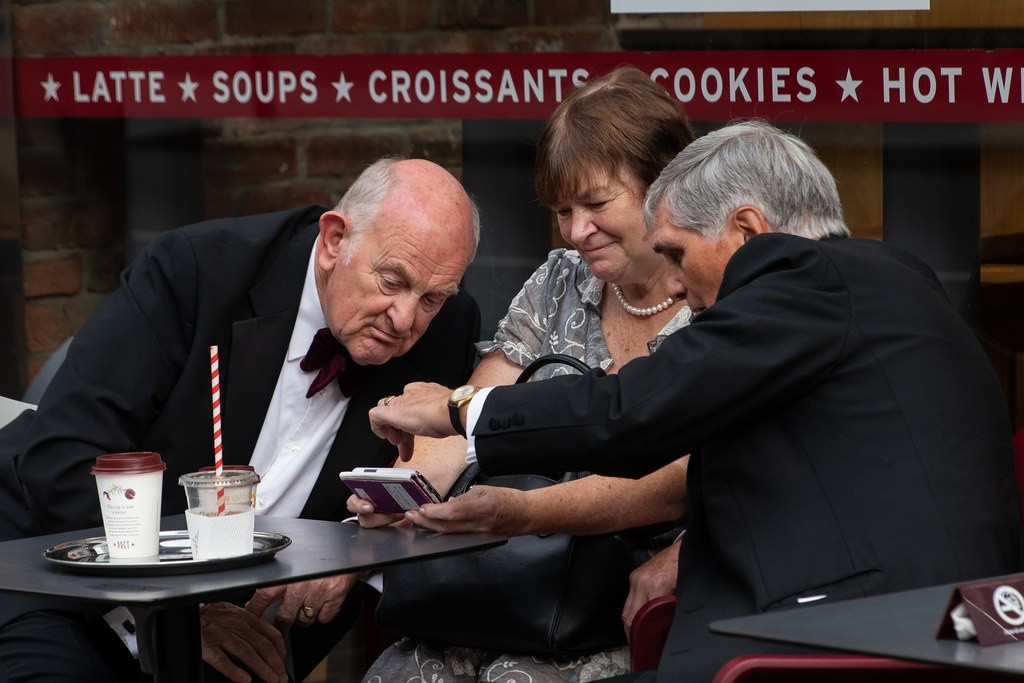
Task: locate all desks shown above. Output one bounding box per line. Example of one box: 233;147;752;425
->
708;573;1024;683
0;513;507;683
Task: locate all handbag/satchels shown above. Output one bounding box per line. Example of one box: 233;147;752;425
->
377;353;639;660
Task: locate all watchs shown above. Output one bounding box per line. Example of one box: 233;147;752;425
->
447;384;483;439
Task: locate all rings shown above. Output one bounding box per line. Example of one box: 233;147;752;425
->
384;396;395;406
302;607;317;618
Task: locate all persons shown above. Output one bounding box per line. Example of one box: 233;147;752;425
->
367;117;1024;683
297;67;703;683
0;156;478;683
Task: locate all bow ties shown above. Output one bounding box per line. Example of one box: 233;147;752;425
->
301;328;374;398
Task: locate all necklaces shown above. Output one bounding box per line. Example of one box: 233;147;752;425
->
612;283;674;317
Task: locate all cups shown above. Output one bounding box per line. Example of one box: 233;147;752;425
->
199;465;254;505
180;471;259;518
90;451;168;557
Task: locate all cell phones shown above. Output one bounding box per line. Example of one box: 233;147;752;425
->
339;467;442;514
